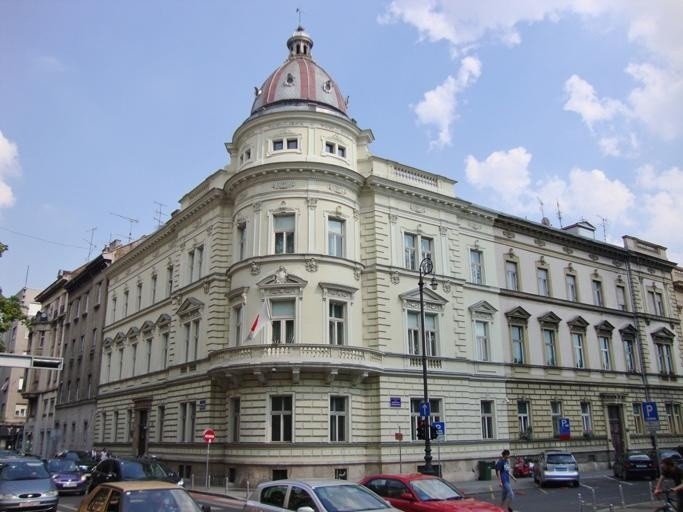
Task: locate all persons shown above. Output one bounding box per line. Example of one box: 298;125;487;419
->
495;449;518;512
653;457;683;512
87;444;113;463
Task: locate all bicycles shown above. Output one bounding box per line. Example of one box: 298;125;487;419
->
652;486;682;512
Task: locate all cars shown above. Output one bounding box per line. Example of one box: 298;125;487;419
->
0;450;97;512
357;473;507;511
657;446;683;459
611;451;656;481
76;480;206;512
88;455;184;497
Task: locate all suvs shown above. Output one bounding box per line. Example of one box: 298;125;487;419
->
534;448;580;488
242;479;403;511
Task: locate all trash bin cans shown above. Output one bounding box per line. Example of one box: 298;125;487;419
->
479;460;492;481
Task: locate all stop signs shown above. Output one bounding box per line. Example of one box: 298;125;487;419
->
203;428;215;442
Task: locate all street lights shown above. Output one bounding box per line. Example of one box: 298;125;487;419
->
416;255;441;474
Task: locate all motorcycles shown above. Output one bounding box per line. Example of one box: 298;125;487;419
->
511;455;535;477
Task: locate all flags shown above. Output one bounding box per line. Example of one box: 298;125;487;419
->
247;297;273;341
